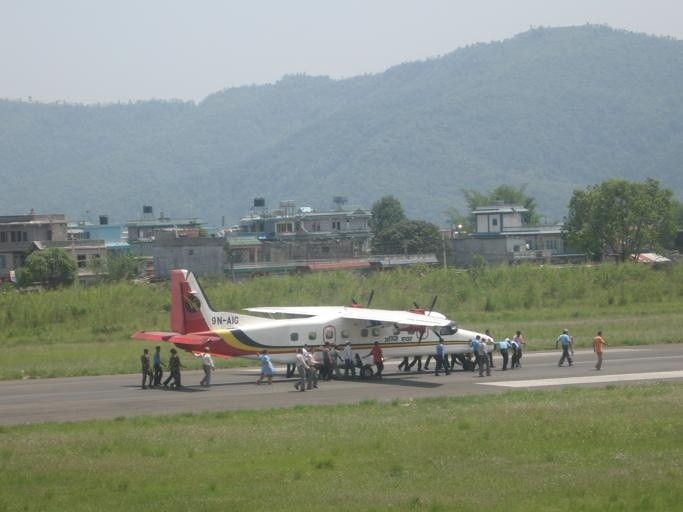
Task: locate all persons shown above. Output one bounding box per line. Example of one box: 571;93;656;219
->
140;348;152;388
152;345;166;385
468;335;523;370
361;340;383;379
294;340;357;392
169;349;183;387
512;330;525;360
434;338;450;376
256;350;273;384
555;327;574;367
485;330;496;368
397;350;477;371
198;346;214;385
592;331;611;370
477;338;491;377
286;363;296;378
162;349;176;386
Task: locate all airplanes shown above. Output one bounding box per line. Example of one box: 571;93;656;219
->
132;268;496;378
